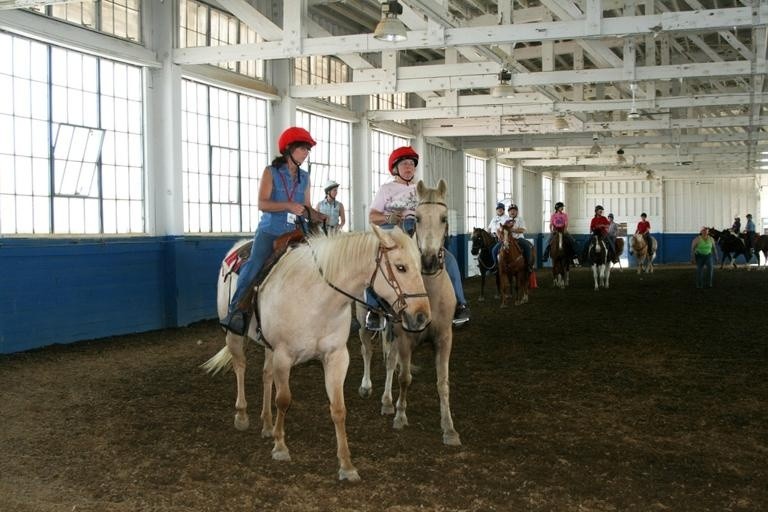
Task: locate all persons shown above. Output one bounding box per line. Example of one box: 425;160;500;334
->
745;214;755;234
220;127;330;336
489;203;534;272
606;214;619;242
487;203;509;240
731;211;745;251
582;205;620;263
628;212;654;255
315;179;346;233
540;202;578;262
690;227;718;288
365;146;468;327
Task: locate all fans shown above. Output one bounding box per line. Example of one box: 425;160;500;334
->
681;169;715;186
659;144;693;167
610;83;672;121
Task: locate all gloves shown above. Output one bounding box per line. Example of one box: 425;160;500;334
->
384;211;403;225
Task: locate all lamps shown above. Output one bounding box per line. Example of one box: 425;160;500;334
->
615;147;628;166
589;133;605;156
490;66;515;100
646;167;657;181
373;0;409;43
552;111;571;132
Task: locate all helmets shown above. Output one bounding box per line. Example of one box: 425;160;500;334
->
389;146;419;176
555;202;563;209
608;214;613;217
323;180;339;193
278;127;316;154
595;206;604;211
508;204;518;212
495;202;505;211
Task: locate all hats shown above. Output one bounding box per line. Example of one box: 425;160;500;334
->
699;224;708;233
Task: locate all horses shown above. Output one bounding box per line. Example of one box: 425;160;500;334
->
629;234;657;275
720;229;768;271
588;227;611;292
708;227;760;269
497;222;536;309
354;178;461;445
197;223;432;482
550;224;571;290
471;227;513;301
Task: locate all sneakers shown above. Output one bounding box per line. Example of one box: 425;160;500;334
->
218;311;246;335
366;309;380;328
455;304;472;319
526;266;532;272
